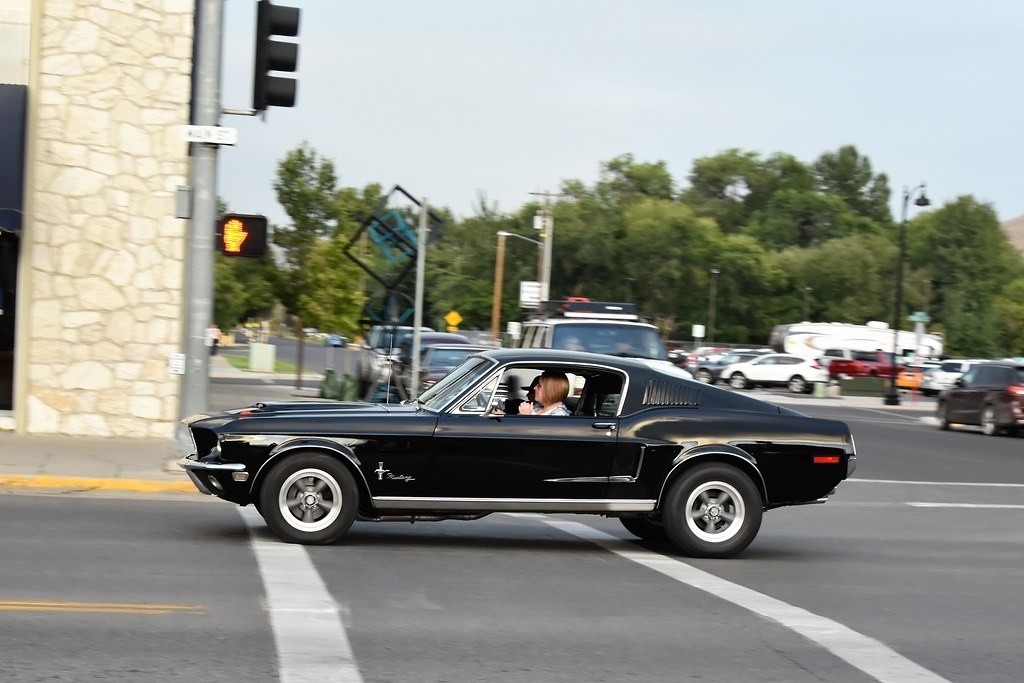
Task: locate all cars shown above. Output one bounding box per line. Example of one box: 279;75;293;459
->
180;347;857;560
357;324;502;411
936;362;1023;436
669;347;1024;394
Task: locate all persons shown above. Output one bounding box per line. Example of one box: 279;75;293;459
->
492;370;573;416
520;376;544;415
564;336;586;351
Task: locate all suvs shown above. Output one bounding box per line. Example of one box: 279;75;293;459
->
491;299;692;410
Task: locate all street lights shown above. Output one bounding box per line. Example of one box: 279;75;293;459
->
884;184;930;406
496;211;554;307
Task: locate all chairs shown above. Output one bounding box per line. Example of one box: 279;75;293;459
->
574;393;620;416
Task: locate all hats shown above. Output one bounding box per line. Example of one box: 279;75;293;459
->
520;376;540;391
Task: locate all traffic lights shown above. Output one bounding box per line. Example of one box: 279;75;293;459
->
219;216;268;258
253;0;300;111
